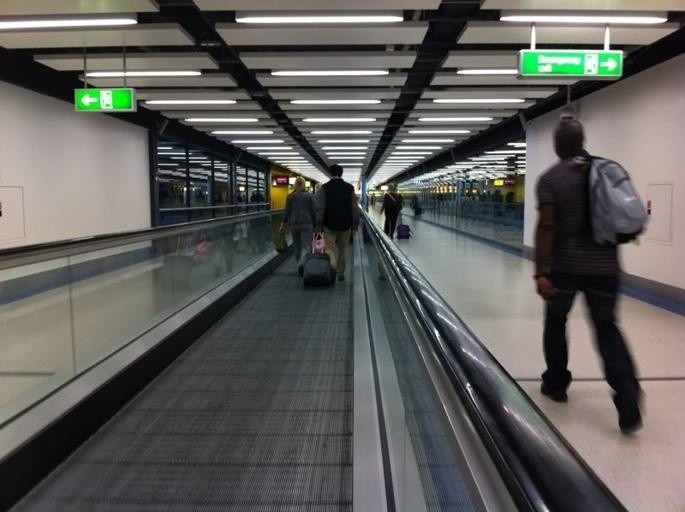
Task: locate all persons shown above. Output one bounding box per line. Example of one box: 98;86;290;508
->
505;186;514;207
234;164;403;281
533;120;642;433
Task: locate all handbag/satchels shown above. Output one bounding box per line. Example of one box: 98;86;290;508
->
277;230;288;253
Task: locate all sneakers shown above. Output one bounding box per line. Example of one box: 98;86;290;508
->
541;383;571;402
337;272;345;281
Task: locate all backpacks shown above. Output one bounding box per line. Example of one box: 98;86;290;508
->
576;150;647;249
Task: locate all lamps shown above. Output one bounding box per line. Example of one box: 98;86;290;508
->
0;1;669;187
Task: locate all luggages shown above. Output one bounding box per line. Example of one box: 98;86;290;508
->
303;232;331;287
397;214;410;240
